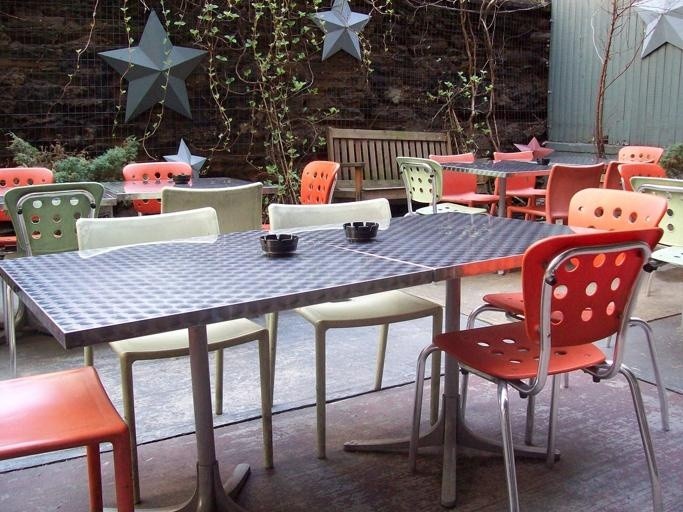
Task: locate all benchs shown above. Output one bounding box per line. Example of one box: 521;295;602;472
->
326;126;452;202
